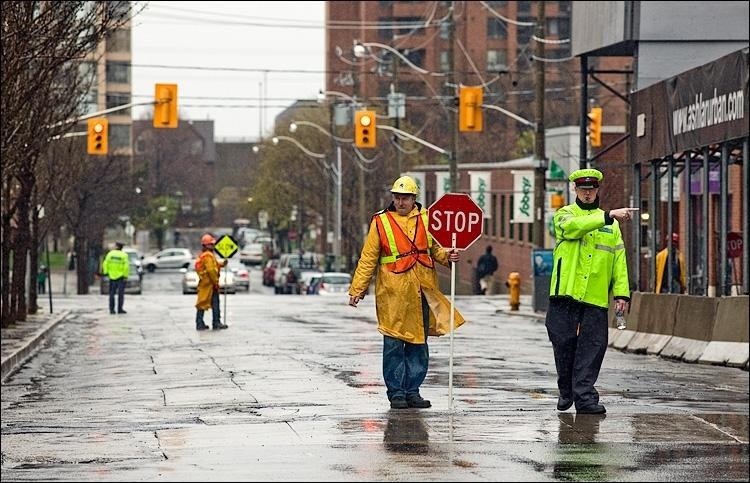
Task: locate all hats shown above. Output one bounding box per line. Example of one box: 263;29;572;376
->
568;168;604;189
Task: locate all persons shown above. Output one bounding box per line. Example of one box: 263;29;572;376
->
478;246;498;295
655;233;686;294
37;265;48;294
103;240;128;314
195;234;228;330
535;253;551;276
544;169;639;414
348;176;461;409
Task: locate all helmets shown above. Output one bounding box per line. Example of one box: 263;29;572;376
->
201;234;216;244
389;175;418;194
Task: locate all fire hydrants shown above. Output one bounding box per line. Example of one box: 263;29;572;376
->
504;270;521;309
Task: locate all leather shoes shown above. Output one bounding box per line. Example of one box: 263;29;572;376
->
118;308;126;313
407;395;431;408
196;322;209;329
213;322;228;329
391;396;408;409
557;394;573;410
576;403;606;413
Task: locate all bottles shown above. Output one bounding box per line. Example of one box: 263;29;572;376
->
615;302;626;330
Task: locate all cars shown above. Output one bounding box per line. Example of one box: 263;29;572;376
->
64;218;356;296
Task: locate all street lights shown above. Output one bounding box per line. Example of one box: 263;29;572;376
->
350;39;459;193
246;88;367;271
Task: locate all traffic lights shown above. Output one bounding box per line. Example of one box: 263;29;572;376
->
354;110;376;146
86;116;108;154
586;107;601;146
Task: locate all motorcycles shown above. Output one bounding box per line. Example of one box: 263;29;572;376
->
466;256;498;296
